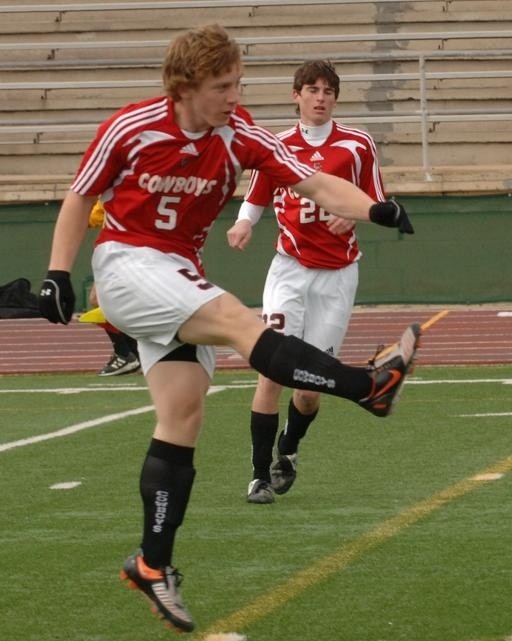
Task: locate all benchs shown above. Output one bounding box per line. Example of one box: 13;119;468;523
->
2;2;512;182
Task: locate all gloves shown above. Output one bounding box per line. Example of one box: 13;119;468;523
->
39;270;75;324
369;200;413;234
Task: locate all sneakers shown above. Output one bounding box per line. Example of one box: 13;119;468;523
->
98;351;139;376
247;430;297;503
358;323;418;416
120;547;193;633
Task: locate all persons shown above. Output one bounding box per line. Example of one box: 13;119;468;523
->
77;187;146;381
37;20;425;634
224;54;402;506
